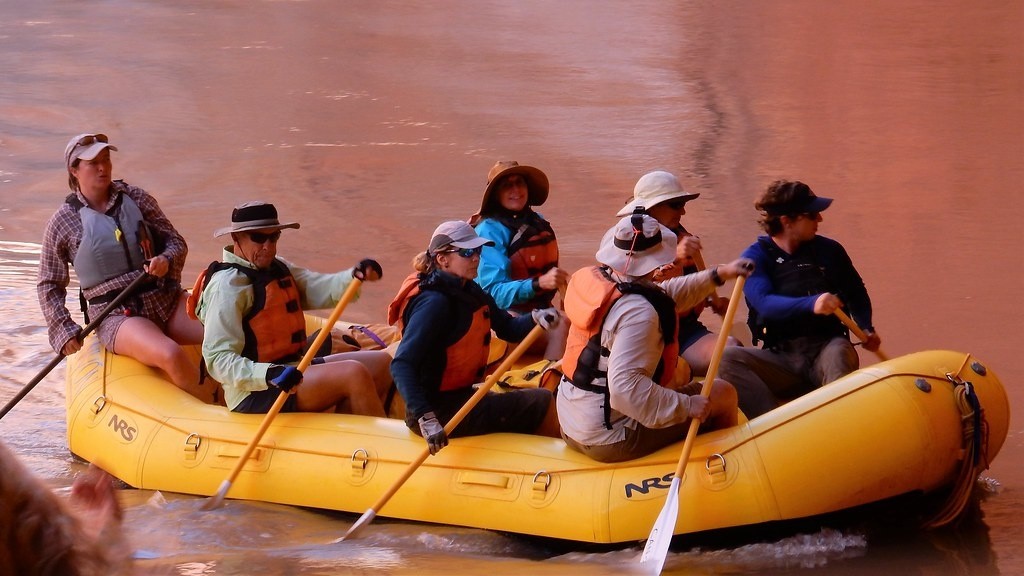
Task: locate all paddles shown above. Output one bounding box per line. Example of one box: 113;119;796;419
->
833;307;891;362
325;323;545;544
196;277;363;511
691;250;725;322
639;262;754;576
0;270;149;420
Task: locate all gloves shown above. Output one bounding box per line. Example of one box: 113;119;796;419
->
418;412;448;456
532;307;561;333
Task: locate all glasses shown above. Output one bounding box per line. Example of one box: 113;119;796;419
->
241;228;281;244
445;246;483;257
661;201;685;210
792;212;818;221
68;134;108;165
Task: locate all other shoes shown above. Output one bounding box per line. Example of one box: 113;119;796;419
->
302;328;332;357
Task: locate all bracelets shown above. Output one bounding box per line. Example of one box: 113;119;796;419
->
711;266;725;286
163;254;174;268
533;277;541;291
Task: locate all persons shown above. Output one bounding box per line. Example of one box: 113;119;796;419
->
195;200;393;418
36;134;227;407
388;220;563;456
466;160;572;361
616;170;744;377
0;438;134;576
556;205;755;464
717;178;881;421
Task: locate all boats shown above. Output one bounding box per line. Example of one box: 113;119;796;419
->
65;314;1009;554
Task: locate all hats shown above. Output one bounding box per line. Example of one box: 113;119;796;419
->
596;214;677;276
429;221;495;257
214;201;300;238
616;171;700;216
761;189;834;215
478;160;549;216
65;133;118;167
